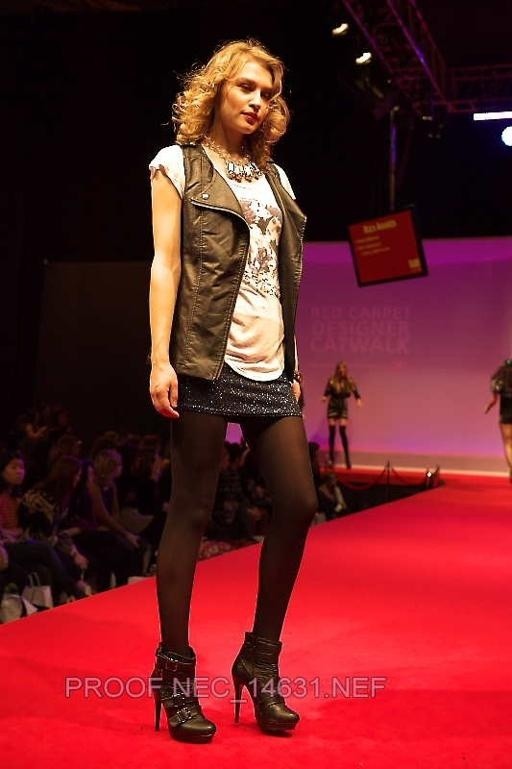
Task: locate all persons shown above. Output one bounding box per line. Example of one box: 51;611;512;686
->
483;358;512;479
148;38;319;746
320;359;362;470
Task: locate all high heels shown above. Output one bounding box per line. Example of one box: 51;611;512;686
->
150;638;217;744
262;717;274;722
229;632;298;733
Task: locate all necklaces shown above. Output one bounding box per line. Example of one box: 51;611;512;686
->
201;130;263;182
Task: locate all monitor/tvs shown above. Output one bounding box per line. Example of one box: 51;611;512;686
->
344;204;429;289
